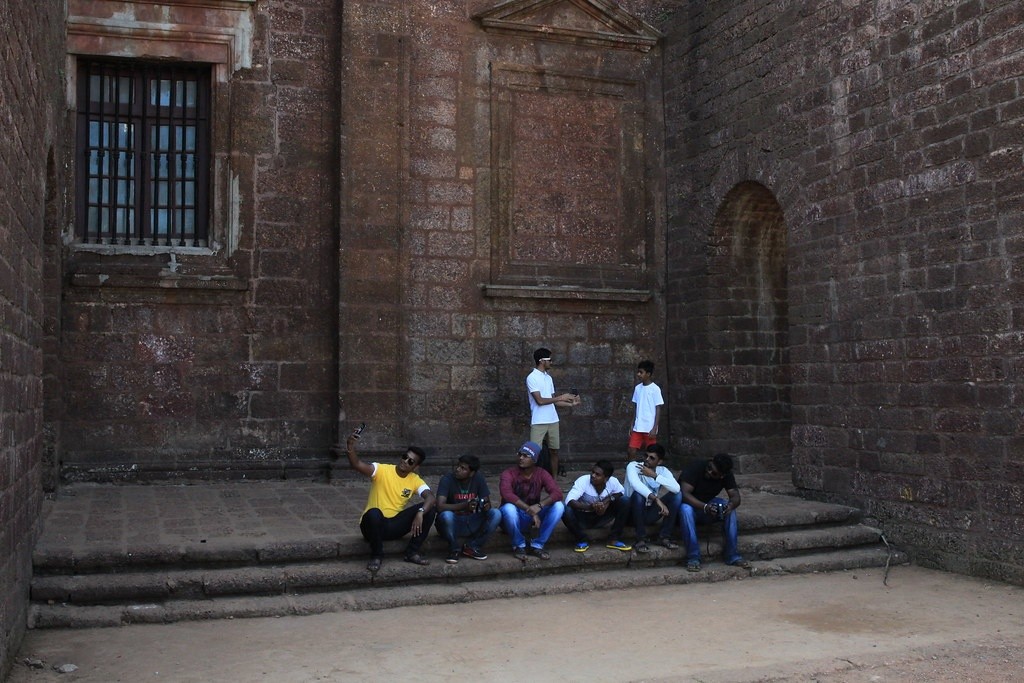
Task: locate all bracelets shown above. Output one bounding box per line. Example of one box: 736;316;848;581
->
704;504;707;514
538;503;543;510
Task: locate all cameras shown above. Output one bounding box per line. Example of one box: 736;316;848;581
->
712;503;728;521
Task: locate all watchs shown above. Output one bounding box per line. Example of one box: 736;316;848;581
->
419;507;424;512
610;495;616;502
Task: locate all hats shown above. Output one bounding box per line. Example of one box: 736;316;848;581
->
518;442;541;460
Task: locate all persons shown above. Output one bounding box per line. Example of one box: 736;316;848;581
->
436;455;502;564
561;460;632;552
498;441;564;560
346;429;436;572
625;444;683;552
627;360;664;463
526;348;581;492
678;453;752;571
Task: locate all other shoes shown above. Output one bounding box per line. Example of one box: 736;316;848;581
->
514;550;528;560
526;546;551;561
688;561;701;572
735;559;751;568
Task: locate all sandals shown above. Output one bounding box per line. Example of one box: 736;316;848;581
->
404;552;430;565
656;536;679;548
635;539;651;553
367;556;382;572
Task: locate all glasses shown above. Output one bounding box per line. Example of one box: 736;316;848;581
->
645;454;658;460
402;454;417;465
538;357;552;362
707;465;721;475
518;451;532;458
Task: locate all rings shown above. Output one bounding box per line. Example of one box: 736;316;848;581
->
417;527;419;528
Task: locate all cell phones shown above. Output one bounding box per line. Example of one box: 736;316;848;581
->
572;388;578;396
354;422;366;439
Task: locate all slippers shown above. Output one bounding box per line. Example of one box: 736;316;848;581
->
607;541;632;550
574;541;590;552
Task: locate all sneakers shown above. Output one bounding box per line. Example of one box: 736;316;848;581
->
462;544;488;560
446;552;461;563
